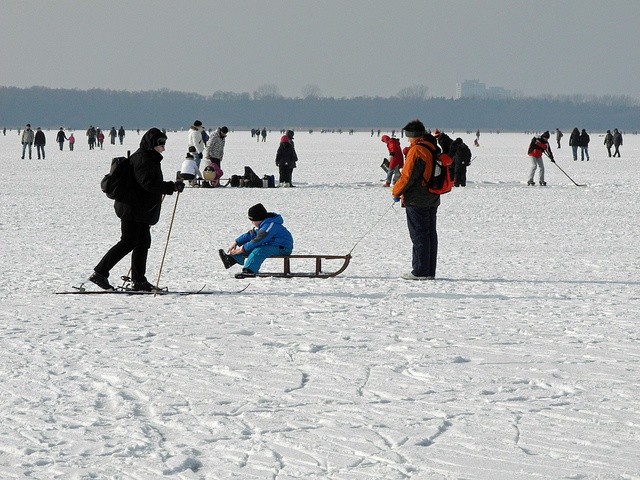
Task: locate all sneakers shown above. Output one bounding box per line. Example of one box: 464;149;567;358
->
235;269;255;278
276;183;284;187
528;181;535;184
282;183;290;187
132;281;160;292
539;181;546;184
401;273;434;280
383;183;390;187
219;249;236;269
88;272;114;291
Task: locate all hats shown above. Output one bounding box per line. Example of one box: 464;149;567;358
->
194;121;202;126
248;204;266;221
150;131;167;147
221;127;228;132
435;130;440;138
186;155;194;160
282;136;288;142
405;121;425;137
542;131;549;138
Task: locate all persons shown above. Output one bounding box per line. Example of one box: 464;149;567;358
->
206;125;229;182
261;127;267;142
602;128;615;158
89;128;184;292
118;124;127;143
67;132;76;148
56;126;68;149
579;127;591;162
109;127;118;144
188;145;202;188
256;127;260;142
35;126;46;158
219;203;294;278
555;127;564;147
570;126;580;159
435;129;454;179
476;129;480;140
391;119;441;281
612;128;622;156
177;153;202;185
381;136;405;188
87;124;105;150
451;137;473;189
19;123;35;159
527;130;555;185
251;127;257;137
18;128;21;135
276;129;299;187
187;120;206;175
3;127;6;136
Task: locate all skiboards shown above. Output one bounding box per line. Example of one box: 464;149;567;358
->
52;284;250;294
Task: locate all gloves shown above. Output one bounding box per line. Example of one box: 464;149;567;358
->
174;181;185;192
391;188;400;202
199;153;203;158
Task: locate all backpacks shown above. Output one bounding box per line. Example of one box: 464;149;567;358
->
416;143;454;195
101;151;142;202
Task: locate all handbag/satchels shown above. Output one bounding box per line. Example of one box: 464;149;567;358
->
287;161;296;169
381;159;394;173
189;146;196;152
210;163;223;179
